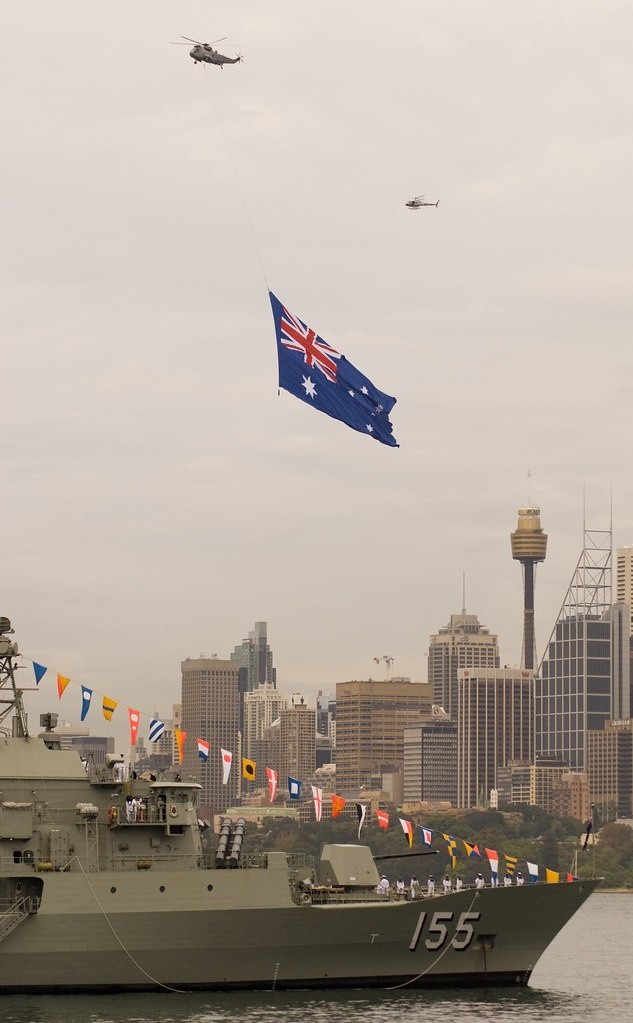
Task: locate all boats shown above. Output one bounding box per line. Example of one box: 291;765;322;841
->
0;615;610;994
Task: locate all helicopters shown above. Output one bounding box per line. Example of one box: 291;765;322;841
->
404;194;440;210
169;34;246;68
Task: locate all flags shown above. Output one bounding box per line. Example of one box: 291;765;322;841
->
149;719;166;743
269;289;399;448
312;786;345;822
462;840;481;858
288;776;301;799
197;738;210;763
102;696;118;721
242;758;256;780
377;811;390;831
221;748;232;784
528;862;579;883
421;827;434;848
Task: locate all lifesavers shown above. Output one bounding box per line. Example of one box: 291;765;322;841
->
108;809;113;821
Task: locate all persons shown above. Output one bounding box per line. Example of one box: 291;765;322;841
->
475;873;524;889
118;754;125;781
443;876;451;893
377;875;389;894
428;875;435;895
82;757;89;771
411;876;419;897
397;876;404;894
457;876;462;891
126;796;141;822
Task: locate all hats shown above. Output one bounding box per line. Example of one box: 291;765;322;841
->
398;876;402;879
413;876;416;878
478;873;482;876
445;875;449;878
518;872;521;875
430;875;434;878
457;875;460;877
505;873;509;876
380;875;386;879
